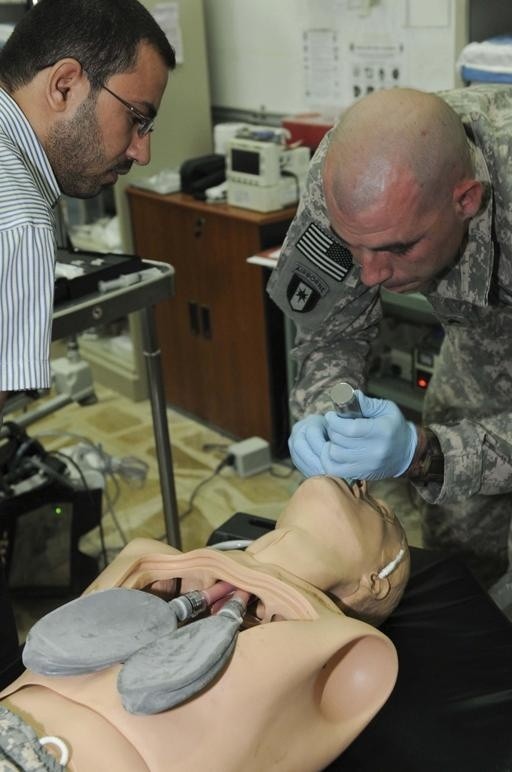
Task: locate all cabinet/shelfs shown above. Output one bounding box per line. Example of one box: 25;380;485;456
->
75;0;299;463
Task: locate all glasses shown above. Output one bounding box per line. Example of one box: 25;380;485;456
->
36;59;154;139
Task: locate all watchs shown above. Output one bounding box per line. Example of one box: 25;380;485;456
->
408;428;447;488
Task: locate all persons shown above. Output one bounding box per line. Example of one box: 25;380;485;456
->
0;0;179;396
0;469;411;772
261;79;509;617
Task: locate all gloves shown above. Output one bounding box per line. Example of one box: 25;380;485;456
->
288;390;418;484
